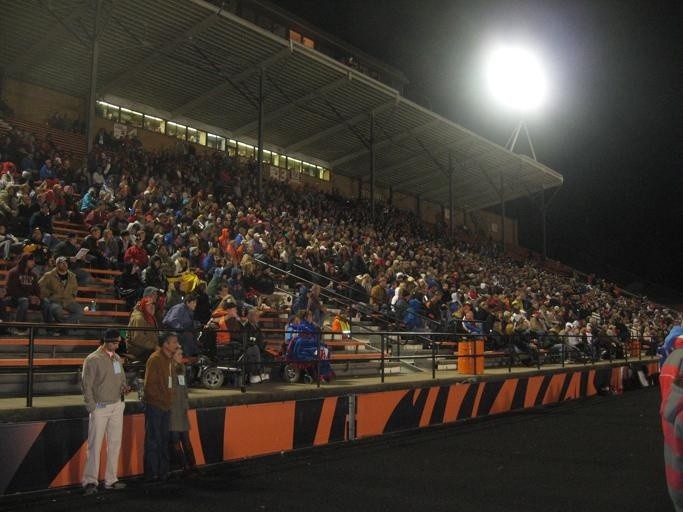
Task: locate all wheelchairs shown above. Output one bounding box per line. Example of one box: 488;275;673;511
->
277;332;334;385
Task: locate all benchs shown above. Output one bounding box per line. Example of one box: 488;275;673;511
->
0;118;510;384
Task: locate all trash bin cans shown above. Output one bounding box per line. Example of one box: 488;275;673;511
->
631;341;640;356
458;339;484;375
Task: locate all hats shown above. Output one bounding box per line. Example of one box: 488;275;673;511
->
223;302;237;310
184;293;199;302
56;256;68;264
103;328;122;343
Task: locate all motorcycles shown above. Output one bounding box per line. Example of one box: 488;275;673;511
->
115;336;224;391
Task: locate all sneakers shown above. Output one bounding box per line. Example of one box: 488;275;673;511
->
105;481;127;490
81;483;98;497
261;372;270;381
250;375;261;383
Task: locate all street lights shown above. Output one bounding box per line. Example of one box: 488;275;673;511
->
494;50;540;161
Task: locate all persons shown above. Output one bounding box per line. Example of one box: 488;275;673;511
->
141;331;197;483
659;331;683;512
0;109;683;381
80;329;128;496
